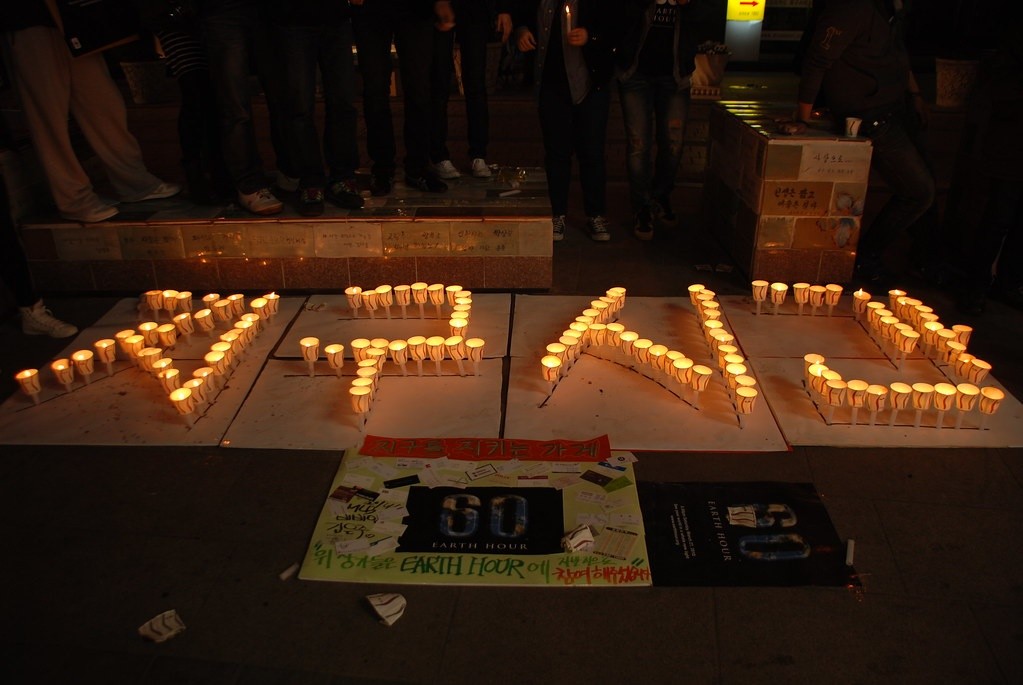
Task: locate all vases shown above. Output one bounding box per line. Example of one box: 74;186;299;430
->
932;58;979;107
693;51;733;87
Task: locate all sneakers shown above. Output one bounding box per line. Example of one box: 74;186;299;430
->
654;195;677;225
238;186;283;214
584;215;610;241
324;182;364;209
552;211;568;240
61;191;119;222
20;299;78;338
121;181;183;202
432;159;460;180
471;157;492;177
404;168;447;193
632;208;654;240
369;164;394;196
291;185;323;215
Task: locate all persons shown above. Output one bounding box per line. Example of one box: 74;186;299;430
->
0;172;80;339
0;0;699;240
773;0;1023;315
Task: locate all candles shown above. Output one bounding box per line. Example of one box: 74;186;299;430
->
301;283;484;430
500;189;521;197
540;285;757;430
751;278;1005;426
845;538;856;565
15;287;280;428
566;13;572;32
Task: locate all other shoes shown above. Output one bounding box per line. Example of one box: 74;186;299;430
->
191;180;219;206
855;253;882;283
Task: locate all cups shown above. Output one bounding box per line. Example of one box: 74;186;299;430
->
799;102;813;121
300;282;485;414
14;289;279;415
846;118;862;138
540;280;1006;416
